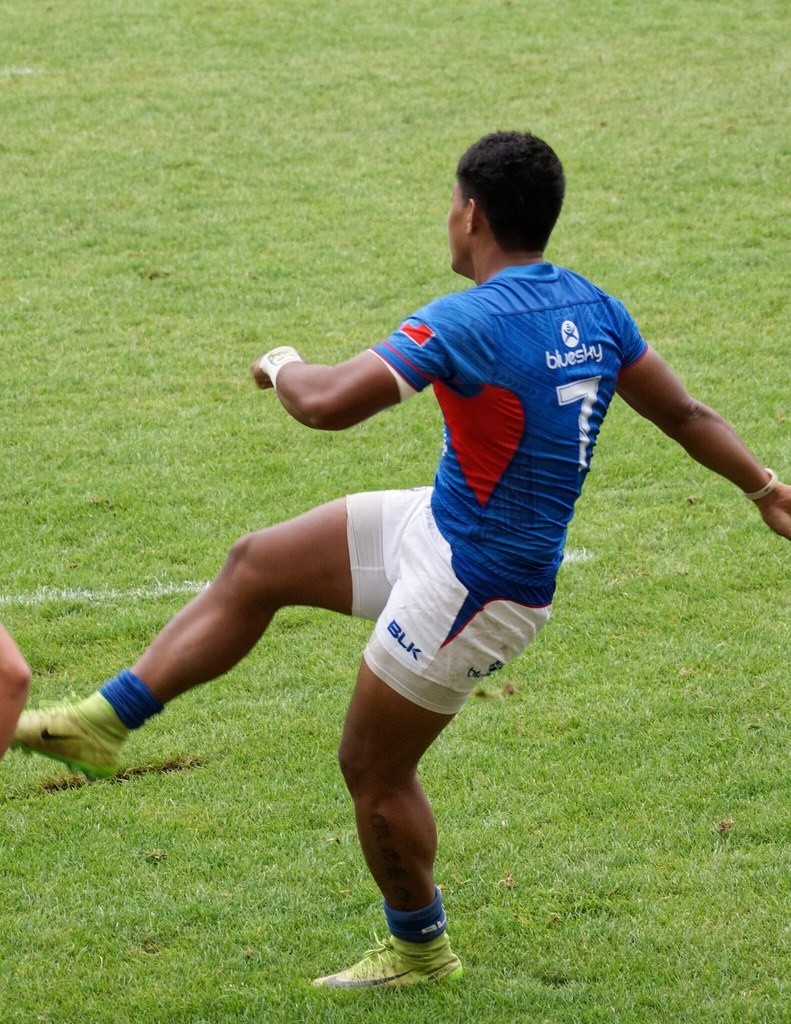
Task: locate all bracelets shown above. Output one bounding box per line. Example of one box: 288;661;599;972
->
745;468;778;500
259;346;304;390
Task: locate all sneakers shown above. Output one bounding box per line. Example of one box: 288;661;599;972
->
307;936;464;996
10;693;125;782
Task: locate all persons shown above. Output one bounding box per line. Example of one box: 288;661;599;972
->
0;623;30;758
8;130;791;991
